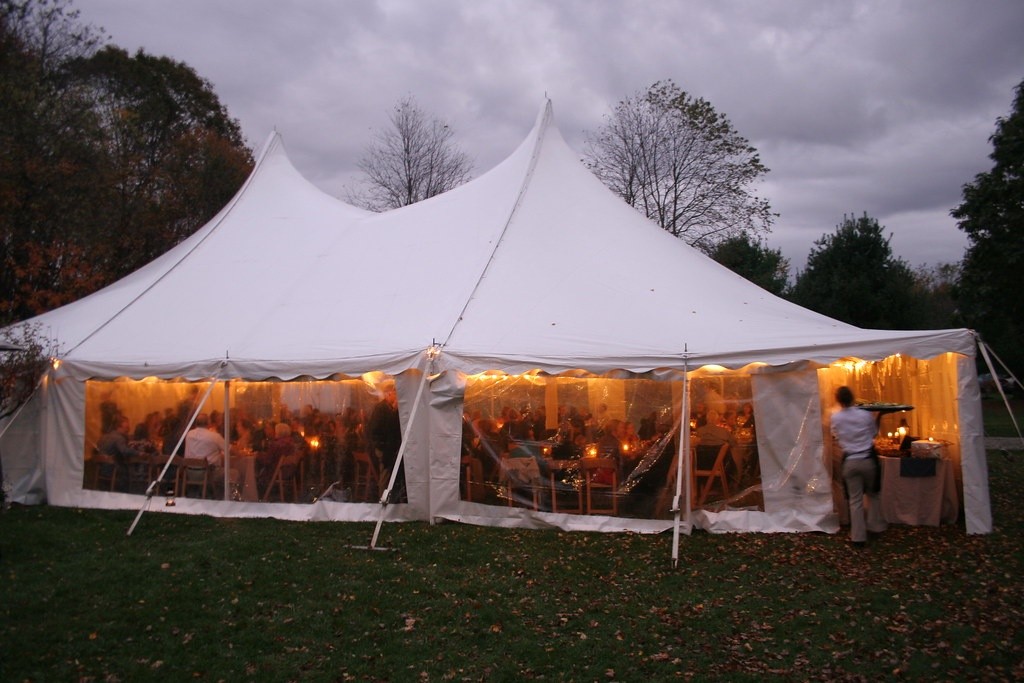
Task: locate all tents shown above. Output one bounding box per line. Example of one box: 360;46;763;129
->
1;99;993;533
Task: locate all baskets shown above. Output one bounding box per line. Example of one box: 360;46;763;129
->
875;444;910;457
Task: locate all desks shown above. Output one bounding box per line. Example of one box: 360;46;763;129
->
871;437;954;524
221;445;259;466
731;437;755;452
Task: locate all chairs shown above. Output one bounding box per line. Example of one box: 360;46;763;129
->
126;456;156;494
352;452;379;501
260;452;303;503
580;458;620;516
501;456;547;513
179;456;218;499
549;459;584;514
154;456;183;497
92;454;129;492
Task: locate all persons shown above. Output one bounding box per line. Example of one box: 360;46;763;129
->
91;386;758;502
831;386;888;544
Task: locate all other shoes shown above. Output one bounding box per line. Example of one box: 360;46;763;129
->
853;541;865;546
